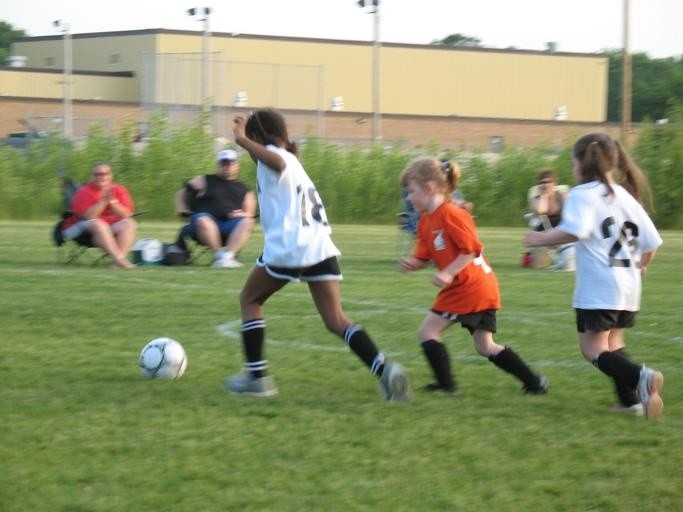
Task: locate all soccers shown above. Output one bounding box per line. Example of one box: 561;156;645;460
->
139;336;187;380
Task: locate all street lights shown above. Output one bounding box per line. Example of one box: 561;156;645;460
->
188;5;213;134
358;0;384;147
53;20;75;141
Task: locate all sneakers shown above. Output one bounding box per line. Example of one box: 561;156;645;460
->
610;402;643;416
637;367;663;418
378;359;410;402
521;375;549;395
225;375;279;398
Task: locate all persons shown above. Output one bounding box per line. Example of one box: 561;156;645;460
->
53;160;136;273
523;168;575;271
394;151;552;395
224;108;409;402
108;199;118;204
523;131;665;424
450;189;473;213
398;192;434;267
171;149;256;271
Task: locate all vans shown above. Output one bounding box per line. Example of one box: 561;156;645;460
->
7;132;31;148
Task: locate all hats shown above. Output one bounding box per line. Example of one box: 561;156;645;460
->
217;149;238;162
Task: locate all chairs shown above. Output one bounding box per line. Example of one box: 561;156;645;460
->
57;176;148;266
174;182;218;266
396;190;464;258
